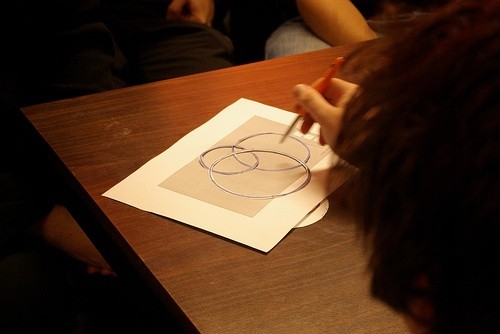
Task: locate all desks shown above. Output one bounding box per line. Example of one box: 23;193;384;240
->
17;33;425;334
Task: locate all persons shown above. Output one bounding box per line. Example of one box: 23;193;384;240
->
264;1;464;60
0;0;246;282
291;0;500;334
